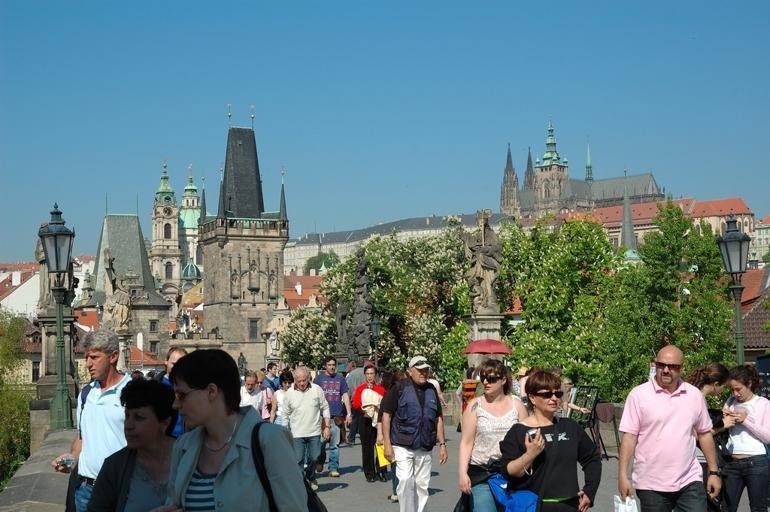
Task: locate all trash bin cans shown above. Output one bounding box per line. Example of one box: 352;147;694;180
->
460;379;478;415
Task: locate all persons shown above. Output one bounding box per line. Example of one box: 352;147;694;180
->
615;345;770;511
457;358;603;510
86;345;447;511
50;329;133;512
465;277;488;314
458;213;506;314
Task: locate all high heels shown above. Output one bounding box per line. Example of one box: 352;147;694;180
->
365;474;374;482
376;473;387;482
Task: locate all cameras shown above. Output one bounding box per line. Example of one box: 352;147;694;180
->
528;428;539;442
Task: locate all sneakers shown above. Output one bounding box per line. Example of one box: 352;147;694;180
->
391;495;398;503
309;464;340;491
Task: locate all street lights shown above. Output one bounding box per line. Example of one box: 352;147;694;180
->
714;210;753;365
37;202;76;430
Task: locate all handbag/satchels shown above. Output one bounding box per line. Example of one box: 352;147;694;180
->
250;420;328;512
613;496;637;512
376;443;395;468
489;474;542;512
65;464;82;512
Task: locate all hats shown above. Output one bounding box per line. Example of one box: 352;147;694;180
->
409;355;432;369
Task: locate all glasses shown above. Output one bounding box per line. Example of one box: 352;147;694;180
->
534;391;563;398
412;360;430;367
480;374;502;383
174;388;198;400
654;362;680;370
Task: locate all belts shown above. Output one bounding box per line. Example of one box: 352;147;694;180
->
81;476;96;486
